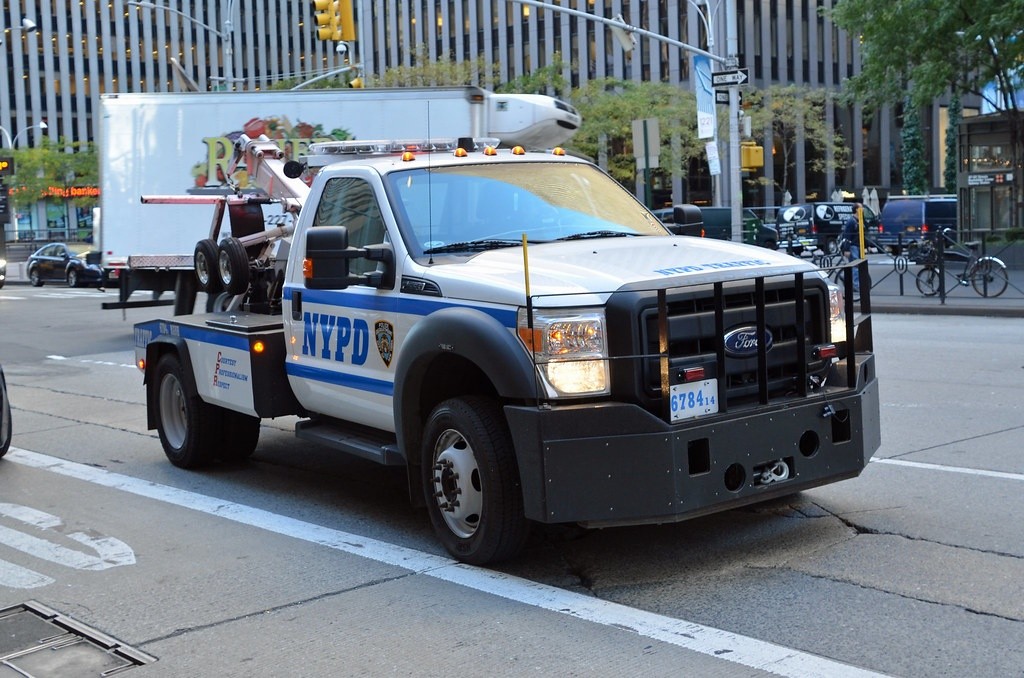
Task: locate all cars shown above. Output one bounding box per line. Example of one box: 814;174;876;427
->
26;242;119;288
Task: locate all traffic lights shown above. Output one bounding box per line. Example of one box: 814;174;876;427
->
348;77;365;90
312;0;339;41
334;0;356;41
767;128;782;156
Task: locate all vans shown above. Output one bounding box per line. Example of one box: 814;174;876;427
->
876;198;958;257
652;207;778;250
776;202;885;258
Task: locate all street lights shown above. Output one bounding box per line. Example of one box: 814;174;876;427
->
0;122;49;239
0;158;15;176
124;1;246;92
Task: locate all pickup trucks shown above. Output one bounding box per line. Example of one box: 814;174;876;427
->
132;136;882;568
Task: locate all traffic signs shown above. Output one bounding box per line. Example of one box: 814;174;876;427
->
711;68;750;86
714;90;742;105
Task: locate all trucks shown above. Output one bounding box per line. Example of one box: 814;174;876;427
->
100;86;596;272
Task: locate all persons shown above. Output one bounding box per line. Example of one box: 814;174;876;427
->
841;202;866;293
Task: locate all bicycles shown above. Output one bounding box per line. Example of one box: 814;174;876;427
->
916;224;1008;298
